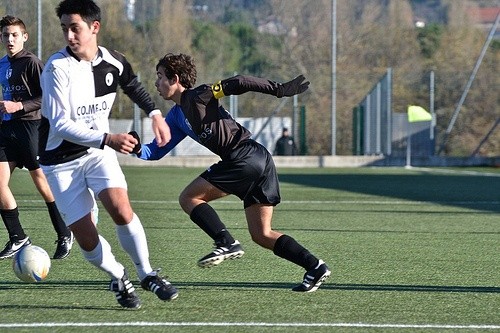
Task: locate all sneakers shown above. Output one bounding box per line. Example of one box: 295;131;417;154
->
197;240;245;269
0;234;31;258
53;226;75;259
293;259;331;292
141;268;180;302
109;263;140;309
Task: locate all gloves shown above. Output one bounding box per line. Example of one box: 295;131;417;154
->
128;130;142;154
277;74;310;98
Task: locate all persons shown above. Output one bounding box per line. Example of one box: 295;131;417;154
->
273;127;296;156
0;15;74;259
38;0;180;311
128;52;331;292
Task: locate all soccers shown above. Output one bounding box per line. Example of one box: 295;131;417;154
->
12;245;50;281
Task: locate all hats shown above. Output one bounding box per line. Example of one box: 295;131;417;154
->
283;128;288;131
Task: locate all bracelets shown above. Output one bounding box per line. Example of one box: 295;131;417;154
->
149;109;162;118
